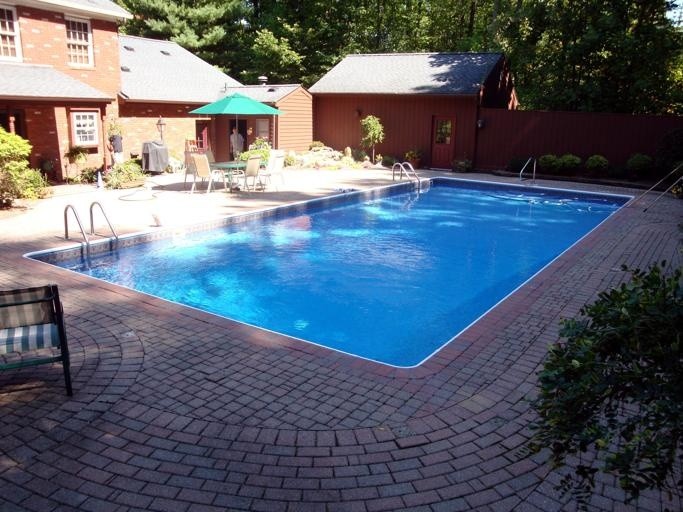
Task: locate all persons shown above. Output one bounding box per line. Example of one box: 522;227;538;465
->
230;126;244;159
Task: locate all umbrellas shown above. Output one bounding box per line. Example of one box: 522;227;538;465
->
188;93;286;153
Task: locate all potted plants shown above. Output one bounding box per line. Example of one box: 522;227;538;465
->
68;146;89;164
404;150;422;169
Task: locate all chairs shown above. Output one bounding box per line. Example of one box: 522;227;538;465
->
182;149;283;194
0;283;73;395
